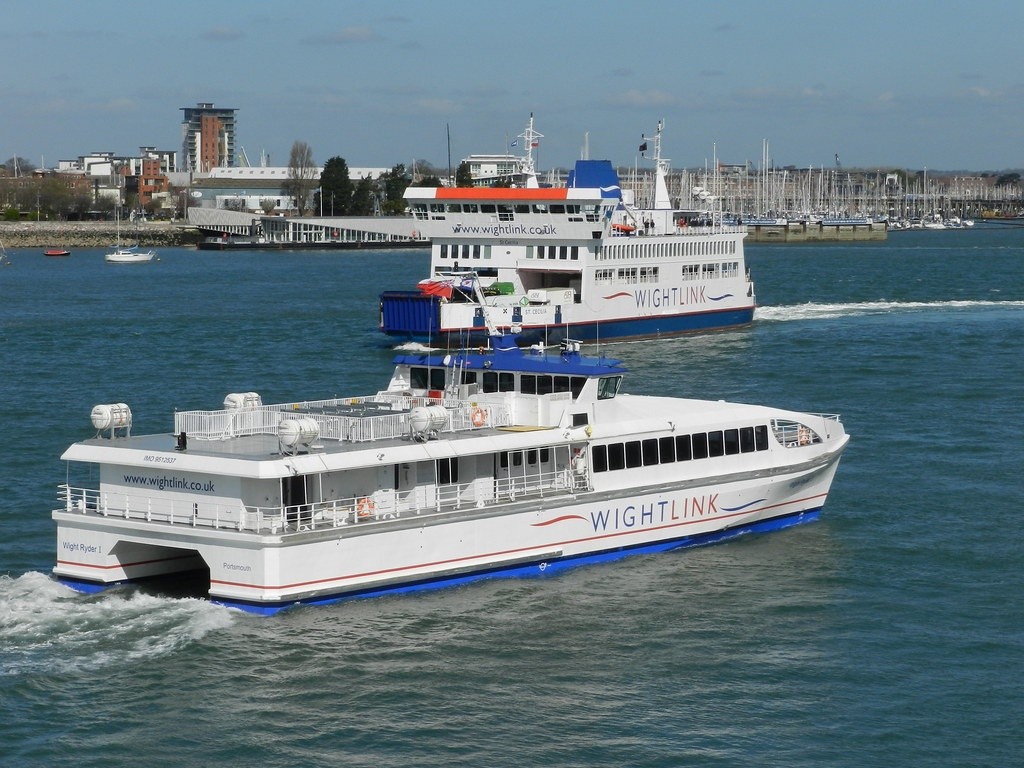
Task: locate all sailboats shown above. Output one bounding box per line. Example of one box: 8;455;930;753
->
621;136;1024;231
105;210;156;261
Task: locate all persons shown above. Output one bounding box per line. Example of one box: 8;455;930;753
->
644;218;655;237
678;215;685;235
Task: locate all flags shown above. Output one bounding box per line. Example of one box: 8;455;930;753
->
531;140;539;147
417;278;454;299
459;277;476;291
638;142;648;151
511;140;517;147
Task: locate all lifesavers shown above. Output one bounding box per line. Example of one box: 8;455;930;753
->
333;232;338;237
612;223;636;231
223;233;227;238
680;218;685;226
412;230;416;236
798;427;811;446
472;408;486;426
357;498;375;516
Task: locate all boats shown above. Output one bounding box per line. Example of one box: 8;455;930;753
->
377;111;758;348
43;248;72;256
612;223;635;231
50;270;850;618
